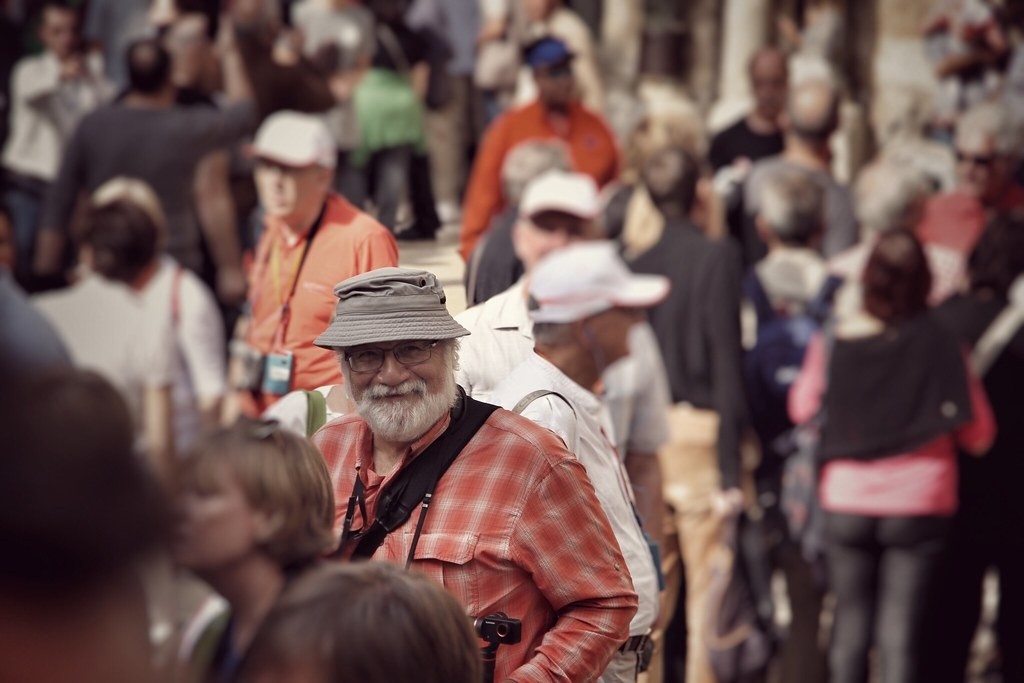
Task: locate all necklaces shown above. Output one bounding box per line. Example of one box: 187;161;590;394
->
598;425;648;535
45;55;83;115
354;419;450;572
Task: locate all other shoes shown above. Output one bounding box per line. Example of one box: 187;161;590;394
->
392;226;437;239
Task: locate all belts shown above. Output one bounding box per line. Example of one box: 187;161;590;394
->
619;633;651;655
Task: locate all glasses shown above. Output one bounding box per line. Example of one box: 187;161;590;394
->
955;152;1004;166
345;339;440;373
212;415;289;454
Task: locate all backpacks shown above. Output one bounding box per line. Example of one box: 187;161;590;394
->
735;275;819;453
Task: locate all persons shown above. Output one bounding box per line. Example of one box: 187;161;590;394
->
0;0;1024;683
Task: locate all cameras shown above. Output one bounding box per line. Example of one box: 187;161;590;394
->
468;612;521;646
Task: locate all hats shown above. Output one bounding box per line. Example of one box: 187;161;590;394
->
312;266;472;350
249;111;338;168
517;167;603;221
527;239;671;325
530;42;576;73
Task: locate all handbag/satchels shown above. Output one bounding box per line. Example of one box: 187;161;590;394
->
351;69;424;147
